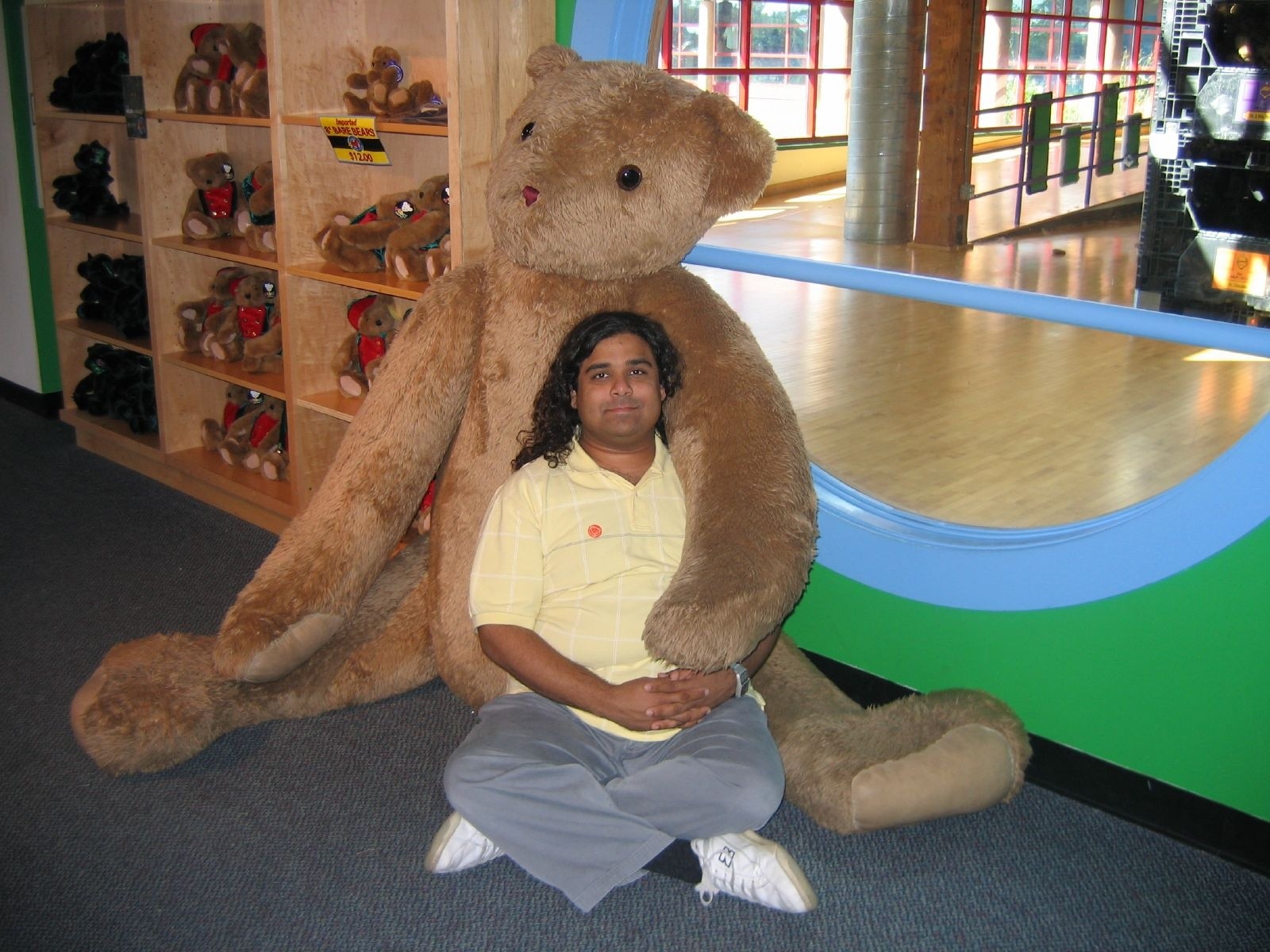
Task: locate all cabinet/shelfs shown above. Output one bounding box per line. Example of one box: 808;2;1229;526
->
0;1;558;561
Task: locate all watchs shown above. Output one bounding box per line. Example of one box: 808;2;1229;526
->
729;659;750;698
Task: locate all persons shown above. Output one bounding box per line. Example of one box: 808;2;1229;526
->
417;312;818;913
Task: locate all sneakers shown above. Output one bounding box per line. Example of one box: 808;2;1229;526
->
690;831;818;913
424;813;505;873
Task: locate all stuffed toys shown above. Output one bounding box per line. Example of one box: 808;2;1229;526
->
44;22;451;543
70;44;1034;835
